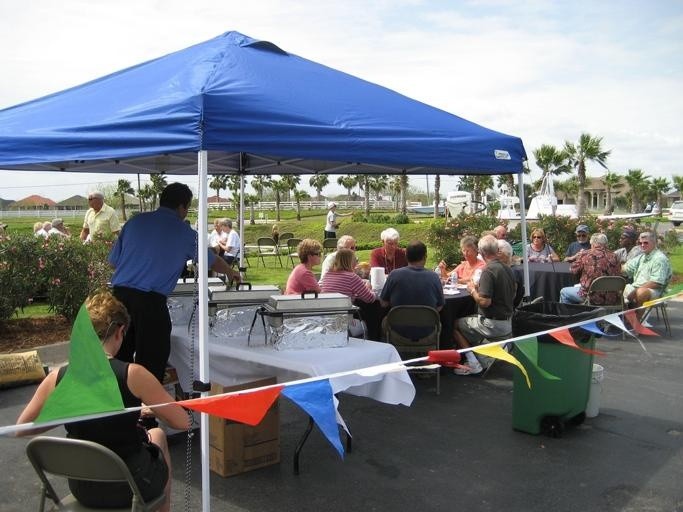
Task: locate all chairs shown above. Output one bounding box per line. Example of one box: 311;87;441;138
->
634;275;674;337
34;339;71;378
345;305;368;338
212;229;349;280
22;436;165;511
475;296;545;379
582;275;628;339
383;306;441;396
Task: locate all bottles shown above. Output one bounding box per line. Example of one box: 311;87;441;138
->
434;265;441;276
450;270;457;290
512;252;554;266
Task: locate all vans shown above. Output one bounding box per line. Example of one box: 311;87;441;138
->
667;201;683;226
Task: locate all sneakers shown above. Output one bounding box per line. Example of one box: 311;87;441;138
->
454;361;483;376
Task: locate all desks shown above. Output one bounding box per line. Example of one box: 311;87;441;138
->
168;322;412;475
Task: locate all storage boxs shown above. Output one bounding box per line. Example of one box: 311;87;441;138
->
206;374;283;477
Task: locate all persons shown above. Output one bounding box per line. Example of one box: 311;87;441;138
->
644;201;660;217
439;225;672;375
272;224;279;253
108;183;242;386
370;228;408;275
318;234;357;287
15;289;190;512
379;239;445;378
324;202;354;239
34;218;71;241
185;218;240;266
284;239;322;295
320;248;380;342
80;191;122;245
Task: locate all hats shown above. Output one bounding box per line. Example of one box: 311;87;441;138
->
576;225;588;233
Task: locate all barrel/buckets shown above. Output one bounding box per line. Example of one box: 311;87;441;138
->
584;362;604;417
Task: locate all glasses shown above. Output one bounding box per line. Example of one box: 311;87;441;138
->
577;234;586;238
533;236;541;239
638;242;648;245
312;252;322;257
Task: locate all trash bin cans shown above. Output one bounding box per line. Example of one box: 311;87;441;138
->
511;302;606;438
